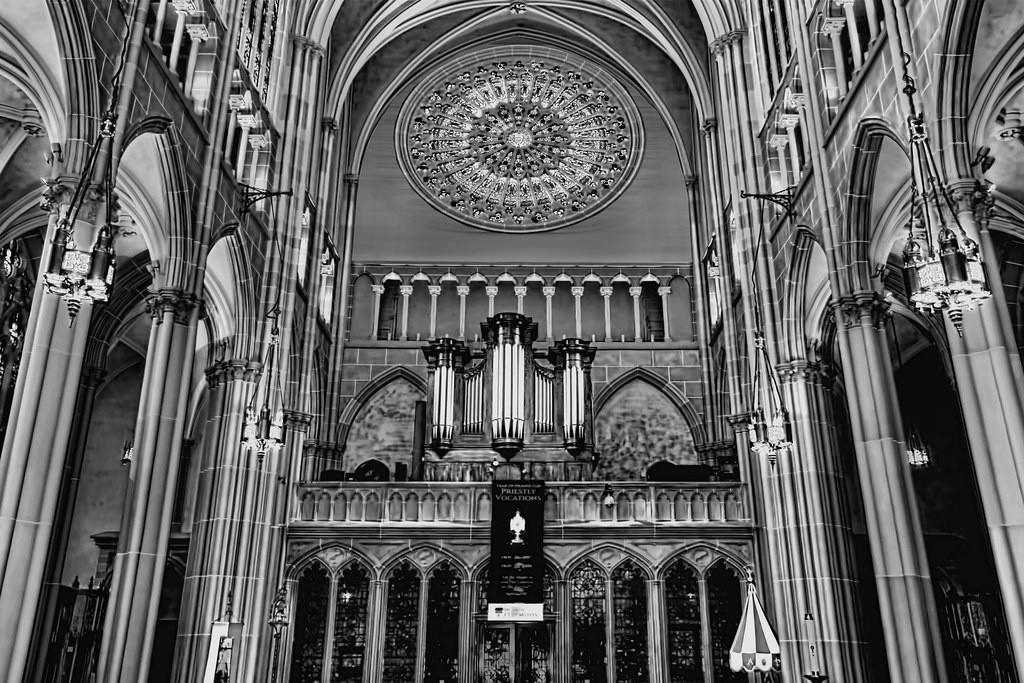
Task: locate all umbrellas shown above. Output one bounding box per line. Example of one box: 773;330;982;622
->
729;582;781;672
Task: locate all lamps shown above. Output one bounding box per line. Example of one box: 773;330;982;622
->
122;440;134;466
40;0;129;327
738;184;794;472
240;183;293;464
891;316;931;471
887;0;997;340
